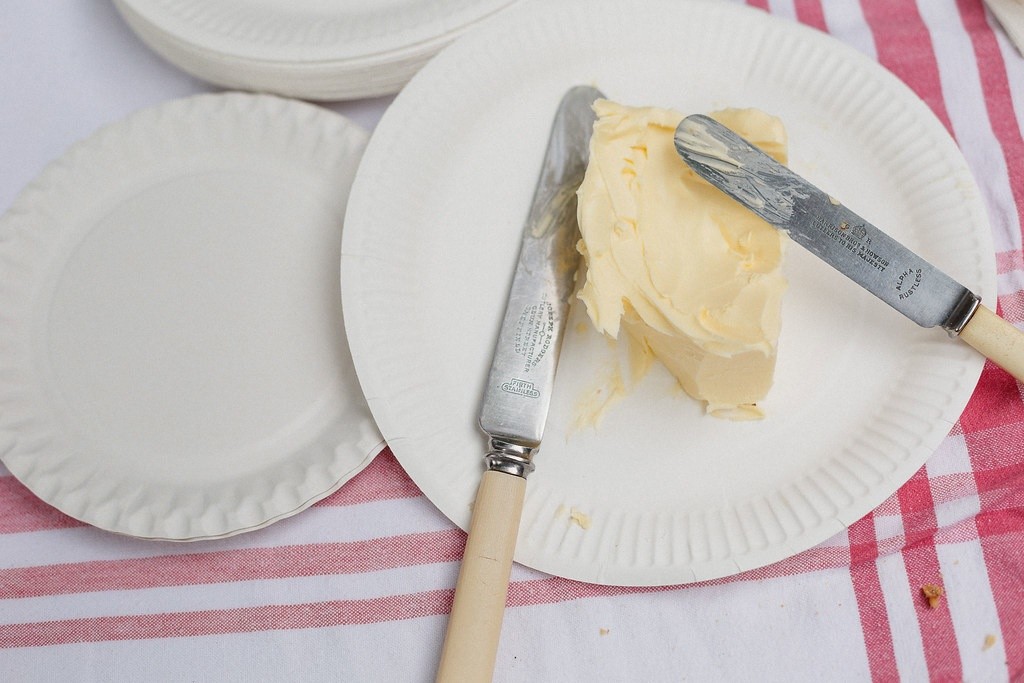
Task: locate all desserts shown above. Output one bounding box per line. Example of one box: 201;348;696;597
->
576;97;786;419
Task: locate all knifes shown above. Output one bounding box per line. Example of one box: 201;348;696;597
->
436;85;609;683
673;115;1024;383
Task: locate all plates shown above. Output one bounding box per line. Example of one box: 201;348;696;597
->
0;0;998;587
